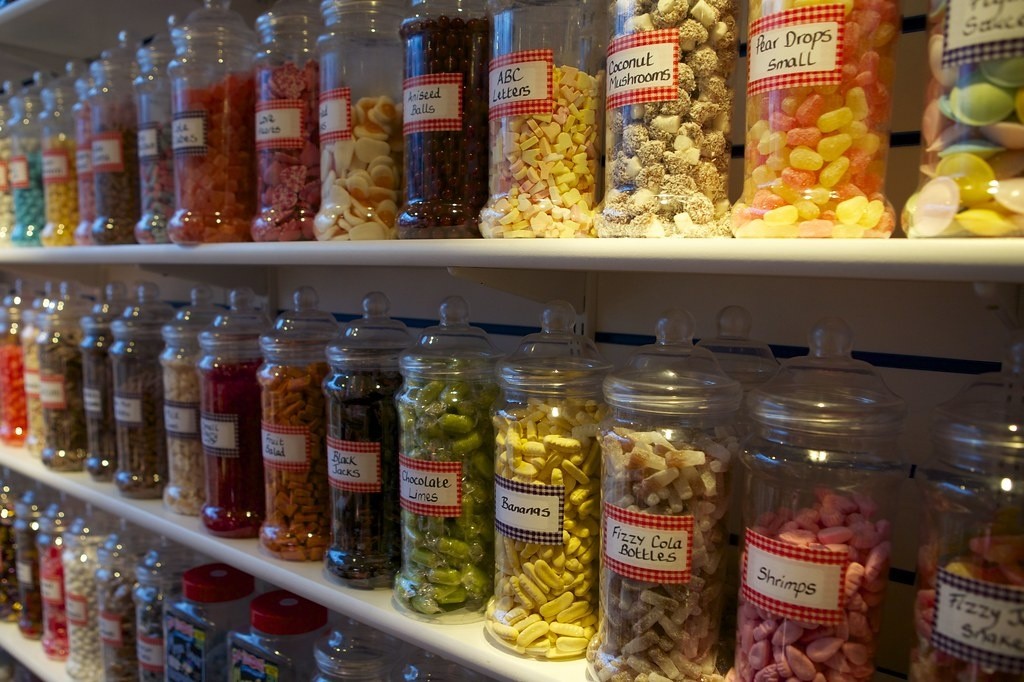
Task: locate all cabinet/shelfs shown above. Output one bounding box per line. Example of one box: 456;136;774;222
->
0;0;1024;682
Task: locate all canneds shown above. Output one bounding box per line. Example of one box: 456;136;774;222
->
0;0;1024;682
